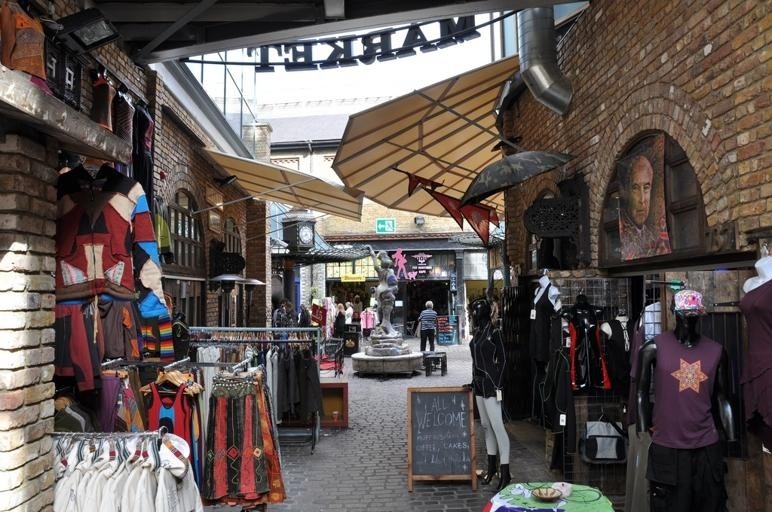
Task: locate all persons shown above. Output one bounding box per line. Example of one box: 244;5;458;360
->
462;298;512;493
161;290;191;361
418;300;440;366
0;0;153;204
619;154;667;264
528;276;634;425
268;287;356;340
359;244;410;334
739;257;772;438
639;290;736;511
529;256;772;511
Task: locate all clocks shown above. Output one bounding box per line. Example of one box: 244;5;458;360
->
298;221;314;246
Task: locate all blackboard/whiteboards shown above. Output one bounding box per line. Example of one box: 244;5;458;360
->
437;315;461;346
407;387;477;480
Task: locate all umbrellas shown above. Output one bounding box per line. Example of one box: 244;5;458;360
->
458;145;578;248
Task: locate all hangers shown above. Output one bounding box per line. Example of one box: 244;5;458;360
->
53;425;177;476
99;360;138;384
141;358;205;396
210;361;270;389
79;154;106;171
190;326;321;361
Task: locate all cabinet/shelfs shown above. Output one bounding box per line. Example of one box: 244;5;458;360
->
318;379;349;427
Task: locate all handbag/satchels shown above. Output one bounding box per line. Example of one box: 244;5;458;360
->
579;414;628;466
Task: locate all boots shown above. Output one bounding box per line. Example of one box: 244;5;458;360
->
481;454;497;485
497;463;510;492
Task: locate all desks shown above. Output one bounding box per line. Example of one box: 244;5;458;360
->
482;480;614;512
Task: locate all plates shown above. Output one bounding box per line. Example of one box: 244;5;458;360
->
533;487;562;502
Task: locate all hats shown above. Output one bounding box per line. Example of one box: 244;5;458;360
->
674;289;706;314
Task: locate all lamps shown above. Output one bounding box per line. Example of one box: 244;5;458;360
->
214;175;238;185
56;9;121;56
238;278;266;292
210;275;246;293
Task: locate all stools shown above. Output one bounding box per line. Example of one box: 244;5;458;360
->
424;351;447;358
425;354;446;376
